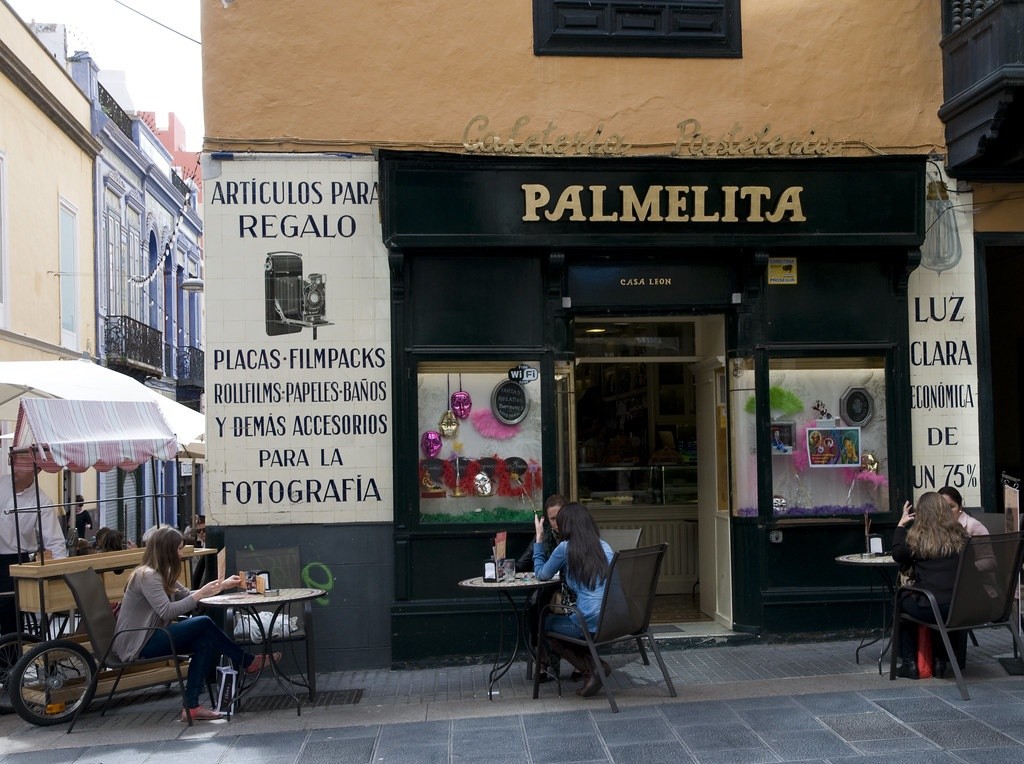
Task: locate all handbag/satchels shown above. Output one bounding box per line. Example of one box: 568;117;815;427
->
554;592;571;615
215;655;238;716
917;624;933;679
110;601;121;620
896;561;918;588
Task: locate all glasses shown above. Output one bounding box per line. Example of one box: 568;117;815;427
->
196;528;205;533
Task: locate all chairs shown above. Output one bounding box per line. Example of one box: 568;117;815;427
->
524;525;644;682
547;544;676;714
863;511;979;645
66;566;214;735
889;532;1024;704
231;547;313;708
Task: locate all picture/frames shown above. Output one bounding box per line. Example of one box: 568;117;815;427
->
771;420;797;456
490;379;530;425
839;386;875;427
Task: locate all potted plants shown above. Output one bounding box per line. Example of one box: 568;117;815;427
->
811;399;839;428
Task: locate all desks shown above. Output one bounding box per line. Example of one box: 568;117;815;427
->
458;571;559;702
199;589;328;722
836;553;898;673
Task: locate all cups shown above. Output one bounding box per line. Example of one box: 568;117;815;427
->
504;559;516;583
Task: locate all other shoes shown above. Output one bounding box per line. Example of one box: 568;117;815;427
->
181;705;223;722
534;664;560;683
571;667;592;682
247;652;279;673
576;661;611;697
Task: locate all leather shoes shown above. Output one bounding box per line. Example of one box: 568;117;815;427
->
895;657;918;679
932;657;946;678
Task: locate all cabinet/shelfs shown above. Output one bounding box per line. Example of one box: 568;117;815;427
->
10;546;217;706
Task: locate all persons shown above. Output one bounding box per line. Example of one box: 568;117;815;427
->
890;486;998;679
64;494;206;570
114;525;282;721
512;494;629;697
0;449;69;683
